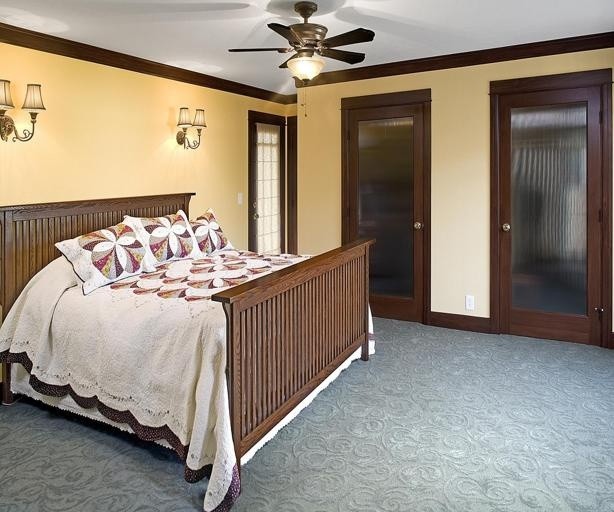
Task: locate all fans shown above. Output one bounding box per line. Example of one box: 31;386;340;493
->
228;1;376;65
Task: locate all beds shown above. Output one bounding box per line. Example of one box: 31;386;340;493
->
1;191;377;492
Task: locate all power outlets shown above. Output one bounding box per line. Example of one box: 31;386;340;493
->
238;193;244;206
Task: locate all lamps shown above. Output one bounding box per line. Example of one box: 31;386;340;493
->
2;79;45;143
175;107;207;150
287;56;325;87
